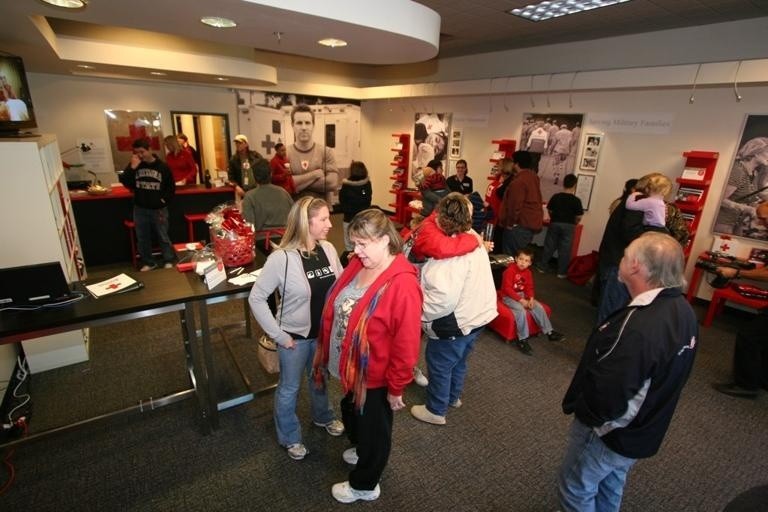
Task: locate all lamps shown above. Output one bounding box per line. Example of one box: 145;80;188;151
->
60;143;91;157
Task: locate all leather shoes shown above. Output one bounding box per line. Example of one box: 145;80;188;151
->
711;383;758;400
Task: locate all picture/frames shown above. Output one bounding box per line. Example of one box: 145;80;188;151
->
710;112;768;245
574;132;604;210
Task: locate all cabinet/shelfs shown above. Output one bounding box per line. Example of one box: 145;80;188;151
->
0;133;91;375
484;139;517;241
389;133;410;224
671;151;720;272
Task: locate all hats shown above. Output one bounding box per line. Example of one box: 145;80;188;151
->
233;135;247;143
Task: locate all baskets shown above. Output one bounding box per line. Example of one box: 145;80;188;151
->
209;226;255;267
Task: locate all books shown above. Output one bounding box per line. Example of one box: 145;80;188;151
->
85;273;145;299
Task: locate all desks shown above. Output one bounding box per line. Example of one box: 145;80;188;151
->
687;250;768;302
1;246;279;449
542;220;583;274
400;188;423;227
69;183;236;266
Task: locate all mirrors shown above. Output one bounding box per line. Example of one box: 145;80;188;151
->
170;110;232;185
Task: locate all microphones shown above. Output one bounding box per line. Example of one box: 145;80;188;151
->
87;170;97;187
115;281;145;295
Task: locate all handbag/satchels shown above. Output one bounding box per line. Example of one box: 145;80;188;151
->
567;251;599;286
256;334;279;374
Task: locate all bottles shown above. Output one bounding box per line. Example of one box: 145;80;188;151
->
205;169;212;188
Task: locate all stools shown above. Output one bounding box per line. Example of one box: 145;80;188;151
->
487;292;552;345
124;220;162;272
704;281;768;327
184;212;209;243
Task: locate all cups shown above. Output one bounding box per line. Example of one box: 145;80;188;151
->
483;224;495;240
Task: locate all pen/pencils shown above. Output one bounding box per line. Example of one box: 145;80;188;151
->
237;268;244;275
228;266;242;275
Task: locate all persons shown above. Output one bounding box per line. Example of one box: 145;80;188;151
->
318;206;424;503
402;190;500;425
412;123;448;161
591;172;689;325
120;138;176;272
240;158;294;244
559;231;698;512
337;159;373;267
165;133;197;187
521;116;581;184
419;148;583;355
269;143;296;197
245;195;344;461
714;137;768;236
284;103;339;212
713;200;767;397
226;134;264;201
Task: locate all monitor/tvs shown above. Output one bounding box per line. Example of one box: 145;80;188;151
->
0;261;73;310
0;55;42;137
66;180;92;191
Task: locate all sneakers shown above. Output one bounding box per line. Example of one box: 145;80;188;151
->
287;367;462;504
548;332;565;342
141;263;158;272
519;339;533;356
163;263;173;269
